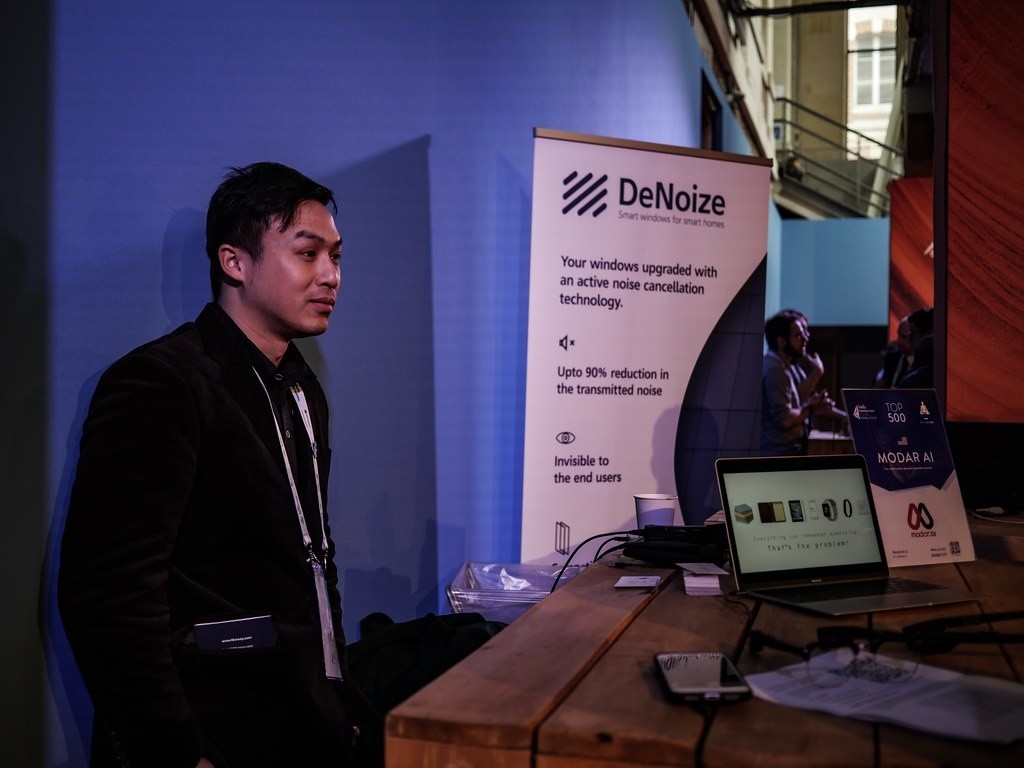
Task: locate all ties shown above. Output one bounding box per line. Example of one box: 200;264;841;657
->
894;357;909;387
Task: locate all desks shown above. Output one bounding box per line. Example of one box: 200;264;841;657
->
385;516;1023;768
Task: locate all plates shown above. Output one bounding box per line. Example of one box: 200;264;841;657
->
973;510;1024;523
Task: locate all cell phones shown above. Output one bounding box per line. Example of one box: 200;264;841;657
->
655;651;753;703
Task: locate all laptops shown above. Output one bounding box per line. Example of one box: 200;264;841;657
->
716;453;985;619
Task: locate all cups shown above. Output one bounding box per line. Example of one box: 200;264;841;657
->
633;494;678;530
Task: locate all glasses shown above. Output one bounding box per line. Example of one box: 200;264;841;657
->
908;329;917;335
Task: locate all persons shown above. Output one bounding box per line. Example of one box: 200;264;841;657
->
56;159;355;768
760;306;936;455
